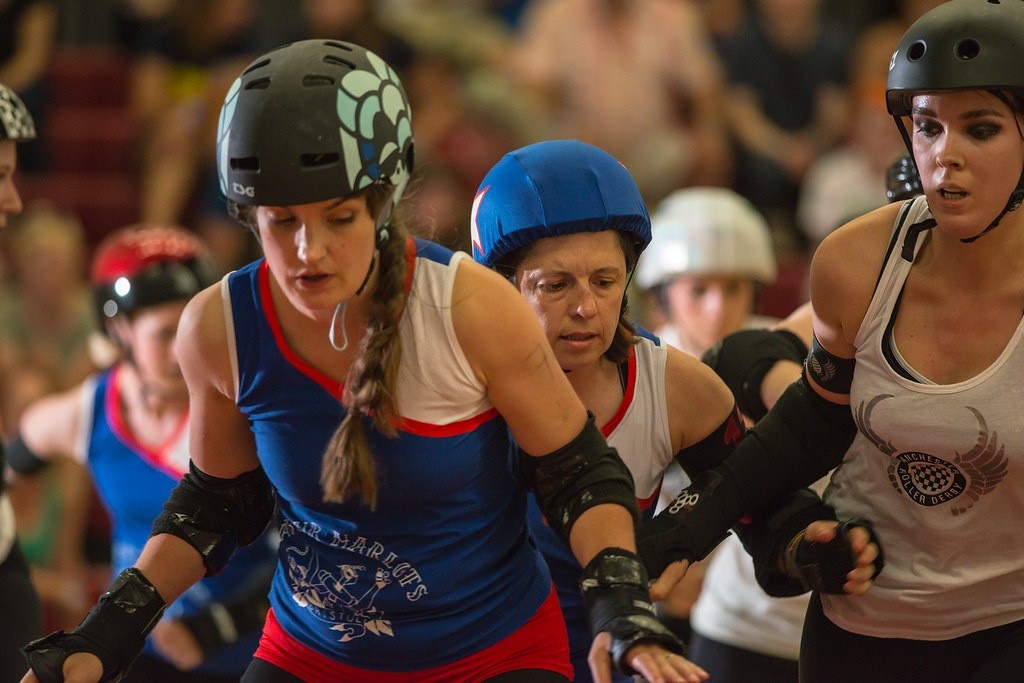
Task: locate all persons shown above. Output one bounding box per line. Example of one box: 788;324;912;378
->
0;0;1024;683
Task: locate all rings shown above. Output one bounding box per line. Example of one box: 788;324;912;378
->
665;653;674;660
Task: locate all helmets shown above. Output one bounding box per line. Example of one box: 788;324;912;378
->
216;38;415;207
795;143;891;242
632;185;778;292
91;224;221;324
885;0;1024;116
0;82;38;140
470;139;652;268
